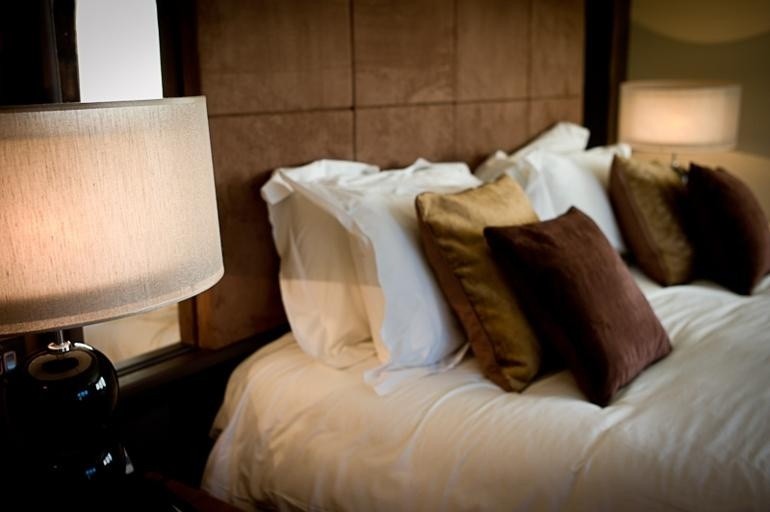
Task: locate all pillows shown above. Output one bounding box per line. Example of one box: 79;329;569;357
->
295;155;502;372
412;170;567;396
663;157;769;298
522;140;636;252
473;119;592;182
480;203;676;408
262;156;383;372
605;154;696;289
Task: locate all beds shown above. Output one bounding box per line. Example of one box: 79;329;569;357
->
200;253;770;511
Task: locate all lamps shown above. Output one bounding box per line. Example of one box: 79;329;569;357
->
1;91;227;512
615;77;743;171
